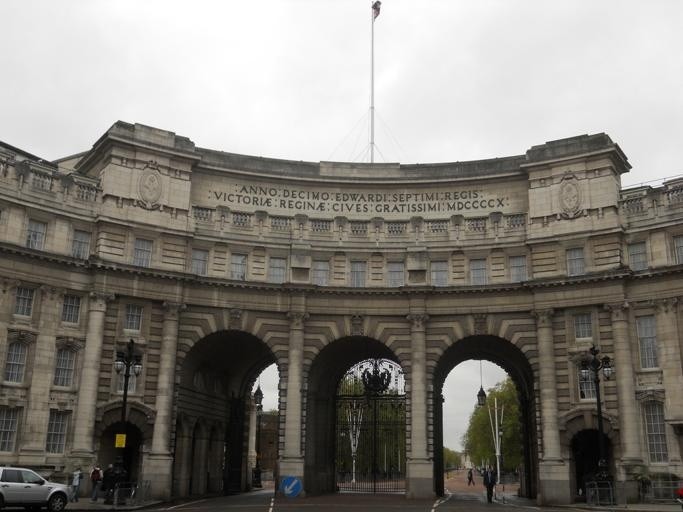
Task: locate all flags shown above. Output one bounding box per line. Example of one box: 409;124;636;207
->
371;1;382;21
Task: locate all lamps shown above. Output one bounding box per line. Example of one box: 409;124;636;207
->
477;359;486;406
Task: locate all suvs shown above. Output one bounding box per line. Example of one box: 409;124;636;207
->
0;465;76;512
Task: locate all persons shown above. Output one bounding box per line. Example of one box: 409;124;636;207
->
467;469;475;486
484;466;496;504
70;463;127;504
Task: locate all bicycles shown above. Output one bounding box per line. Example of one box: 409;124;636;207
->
127;473;147;505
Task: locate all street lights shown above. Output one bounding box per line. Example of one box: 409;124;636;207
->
463;451;467;467
497;421;505;483
110;333;145;507
251;383;265;490
575;340;614;483
339;429;346;462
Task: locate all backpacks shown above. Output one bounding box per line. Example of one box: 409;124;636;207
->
90;468;101;483
